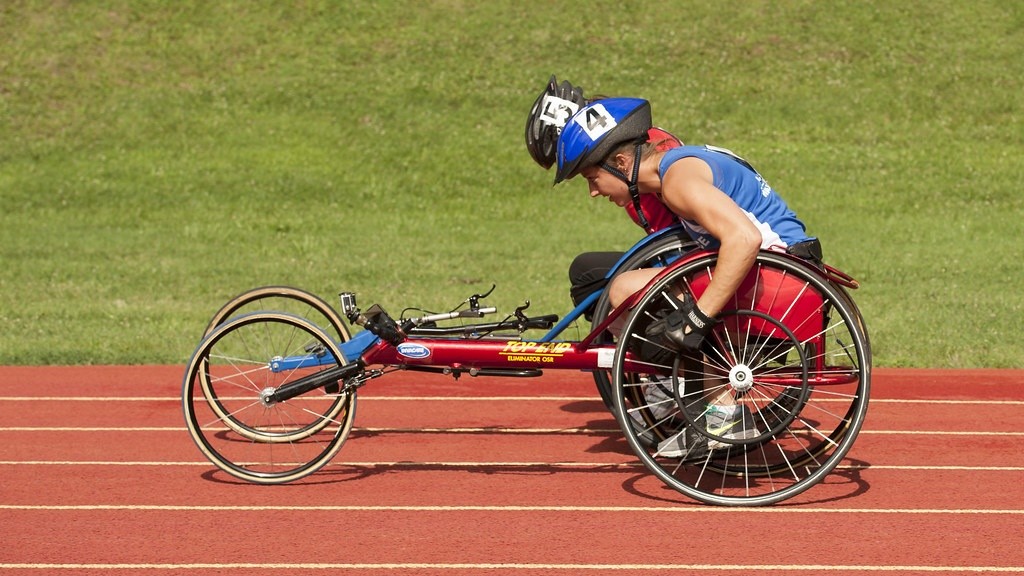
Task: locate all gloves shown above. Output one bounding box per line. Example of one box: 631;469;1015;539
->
645;301;724;351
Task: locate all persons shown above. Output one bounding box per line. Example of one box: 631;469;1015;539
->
523;75;824;457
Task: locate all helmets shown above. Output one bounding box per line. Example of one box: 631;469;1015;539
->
552;99;648;188
525;74;589;171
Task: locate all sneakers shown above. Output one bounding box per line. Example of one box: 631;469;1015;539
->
630;375;685;426
657;404;761;458
